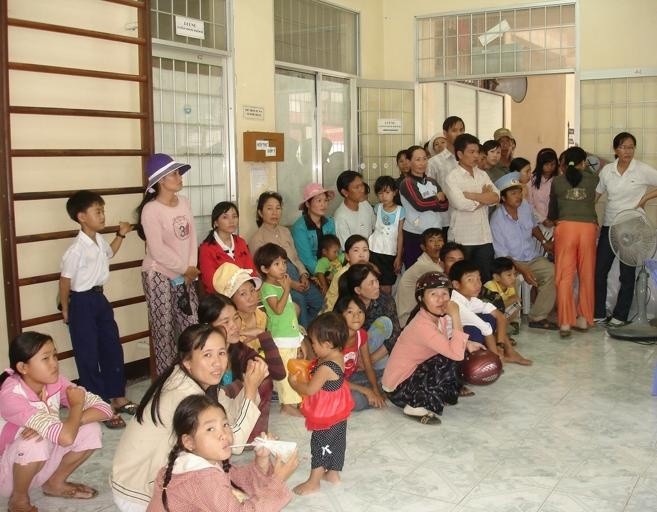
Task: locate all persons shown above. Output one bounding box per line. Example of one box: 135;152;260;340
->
288;311;356;495
197;169;406;429
0;329;114;511
397;116;559;366
58;191;143;431
143;395;300;512
381;271;479;425
107;322;269;511
548;132;657;335
135;153;200;377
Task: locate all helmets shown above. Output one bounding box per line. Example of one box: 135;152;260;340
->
415;271;454;301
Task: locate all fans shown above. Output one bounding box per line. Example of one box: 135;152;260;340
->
607;209;657;338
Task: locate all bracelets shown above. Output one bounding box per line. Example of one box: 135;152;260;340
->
114;231;125;238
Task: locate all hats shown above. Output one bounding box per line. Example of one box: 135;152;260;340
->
494;172;523;194
495;129;512;141
145;154;191;195
299;184;334;210
427;134;448;157
213;262;262;298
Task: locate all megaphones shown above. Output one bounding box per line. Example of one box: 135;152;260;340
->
492;75;528;103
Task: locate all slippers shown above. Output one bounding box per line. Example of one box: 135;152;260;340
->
403;411;441;425
6;498;36;512
497;318;625;348
113;401;138;415
43;483;98;499
103;412;126;428
